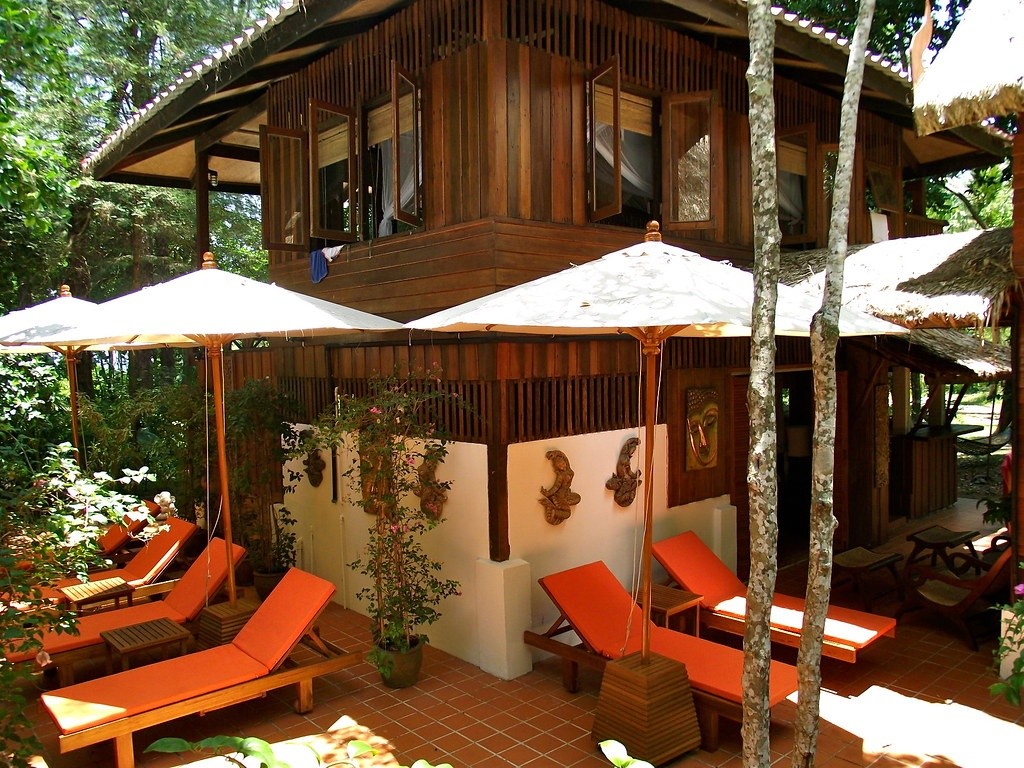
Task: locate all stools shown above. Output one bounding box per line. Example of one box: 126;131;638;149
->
833;543;905;614
905;525;982;576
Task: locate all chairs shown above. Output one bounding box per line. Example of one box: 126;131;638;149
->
945;535;1011;576
891;542;1013;652
0;516;202;619
522;560;798;753
0;537;249;689
652;530;896;674
37;566;363;768
0;499;163;579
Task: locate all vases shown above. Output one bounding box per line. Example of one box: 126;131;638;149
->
373;635;423;688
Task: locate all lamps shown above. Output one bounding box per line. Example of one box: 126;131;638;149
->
207;168;218;187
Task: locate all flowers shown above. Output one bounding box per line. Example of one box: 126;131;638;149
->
286;358;485;680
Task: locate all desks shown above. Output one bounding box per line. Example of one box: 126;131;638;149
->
60;577;135;619
99;617;191;675
628;582;704;638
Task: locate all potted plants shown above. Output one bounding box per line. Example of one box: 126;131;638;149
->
231;450;304;603
186;392;263;588
